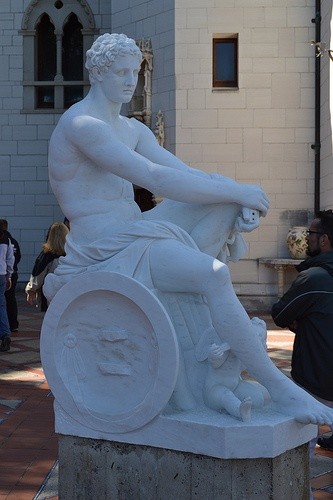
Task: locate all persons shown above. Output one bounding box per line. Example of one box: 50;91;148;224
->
203;317;267;422
24;223;70;312
48;33;333;426
0;227;15;352
0;219;21;331
271;209;333;450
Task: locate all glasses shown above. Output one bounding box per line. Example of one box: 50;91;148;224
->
305;229;319;235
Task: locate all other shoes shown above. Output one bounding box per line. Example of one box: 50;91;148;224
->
11;321;19;331
316;433;333;451
0;335;11;352
